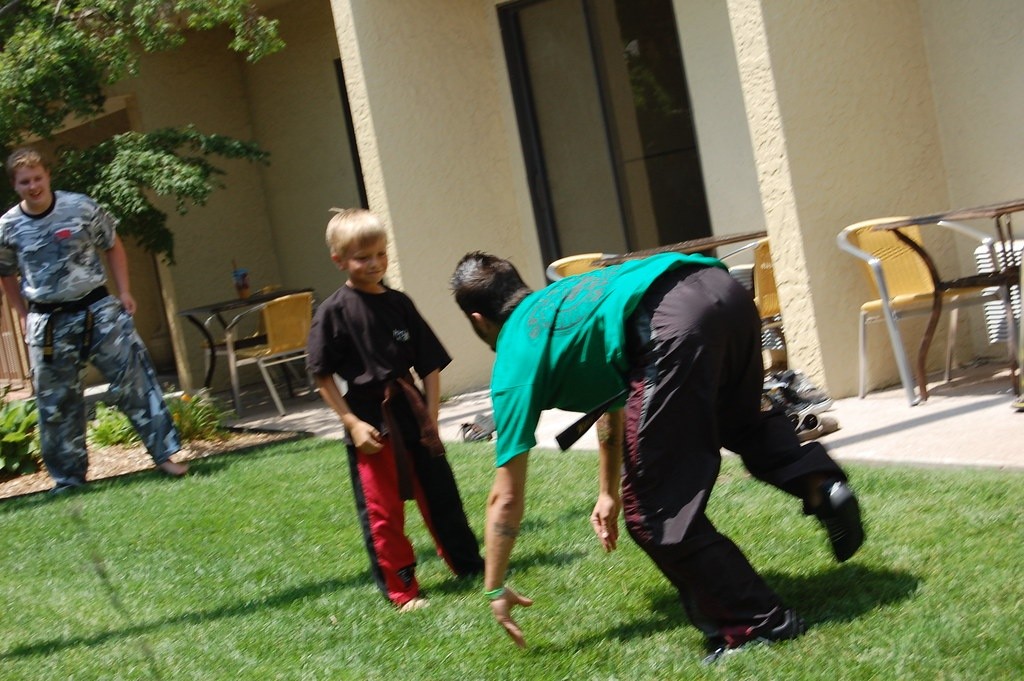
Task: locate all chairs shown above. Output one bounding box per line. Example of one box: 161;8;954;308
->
202;292;315;417
836;216;1019;407
753;238;788;380
546;252;625;283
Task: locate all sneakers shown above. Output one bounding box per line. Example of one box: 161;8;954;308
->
821;480;865;565
702;609;797;653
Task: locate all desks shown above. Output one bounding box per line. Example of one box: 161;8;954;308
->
867;196;1024;412
175;286;315;399
588;228;767;270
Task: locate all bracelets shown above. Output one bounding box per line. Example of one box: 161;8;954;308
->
484;584;505;599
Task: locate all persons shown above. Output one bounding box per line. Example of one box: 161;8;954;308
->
450;249;867;666
307;208;510;612
0;146;188;496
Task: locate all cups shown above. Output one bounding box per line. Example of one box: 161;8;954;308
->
231;268;252;299
